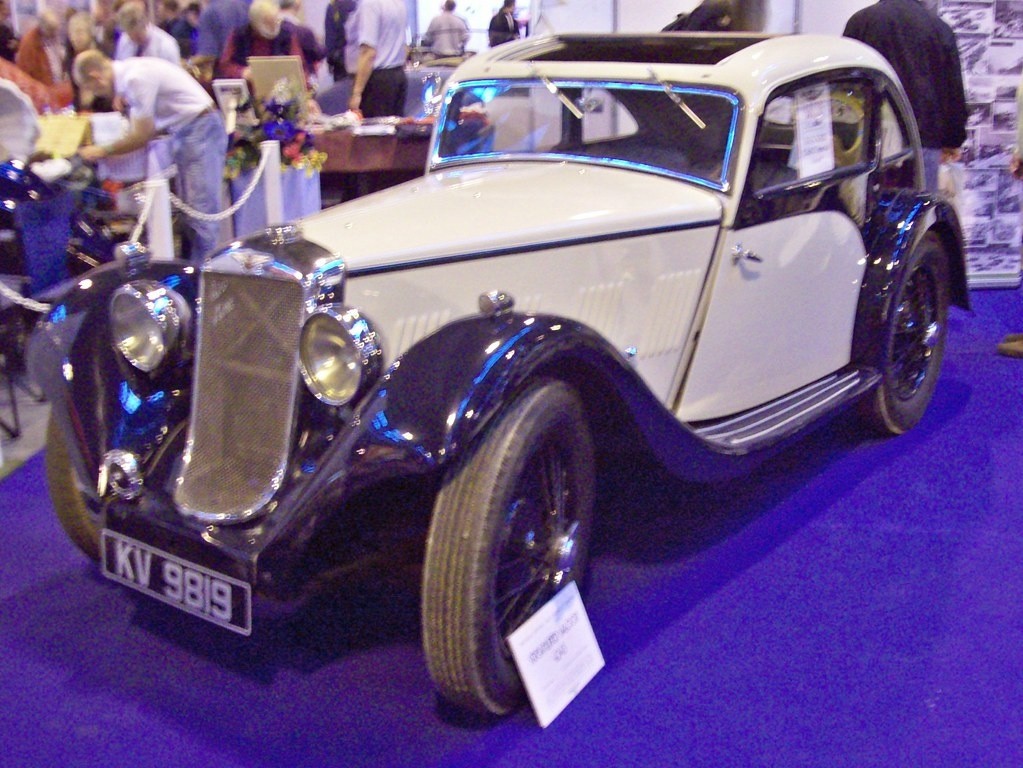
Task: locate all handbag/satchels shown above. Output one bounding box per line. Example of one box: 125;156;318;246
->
938;160;966;208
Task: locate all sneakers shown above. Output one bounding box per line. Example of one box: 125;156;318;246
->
995;331;1023;360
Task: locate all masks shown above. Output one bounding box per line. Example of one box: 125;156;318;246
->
258;23;281;40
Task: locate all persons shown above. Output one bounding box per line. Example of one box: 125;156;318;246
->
1;0;529;266
994;73;1023;358
842;0;970;195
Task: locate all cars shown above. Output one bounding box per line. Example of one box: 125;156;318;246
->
29;27;970;715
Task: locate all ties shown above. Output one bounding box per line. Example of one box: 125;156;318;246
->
136;44;143;57
